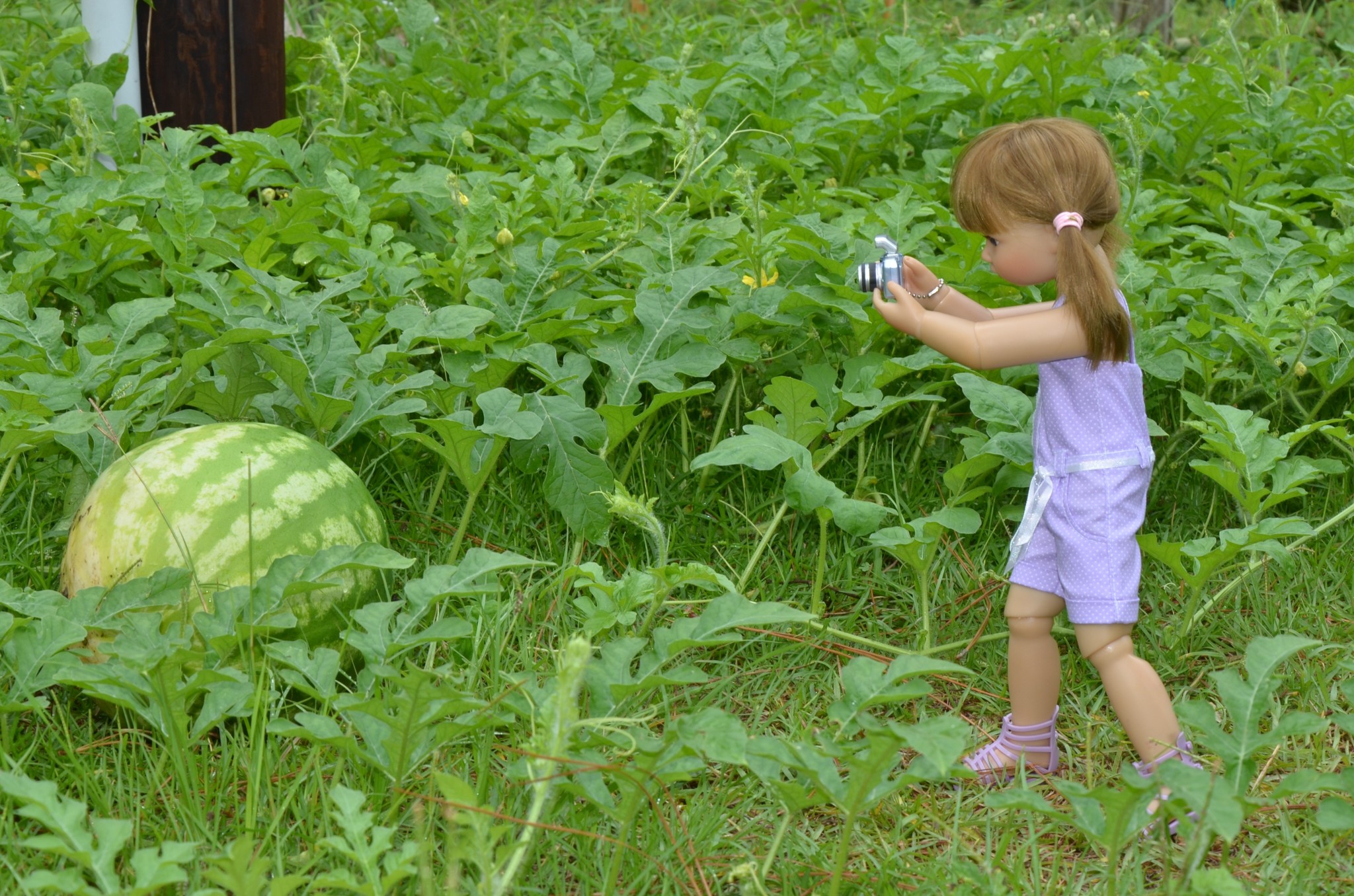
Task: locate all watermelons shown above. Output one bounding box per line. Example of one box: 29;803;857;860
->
59;420;395;731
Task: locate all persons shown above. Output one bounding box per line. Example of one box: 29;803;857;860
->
870;118;1205;839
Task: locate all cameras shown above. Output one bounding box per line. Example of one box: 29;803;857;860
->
857;236;904;299
